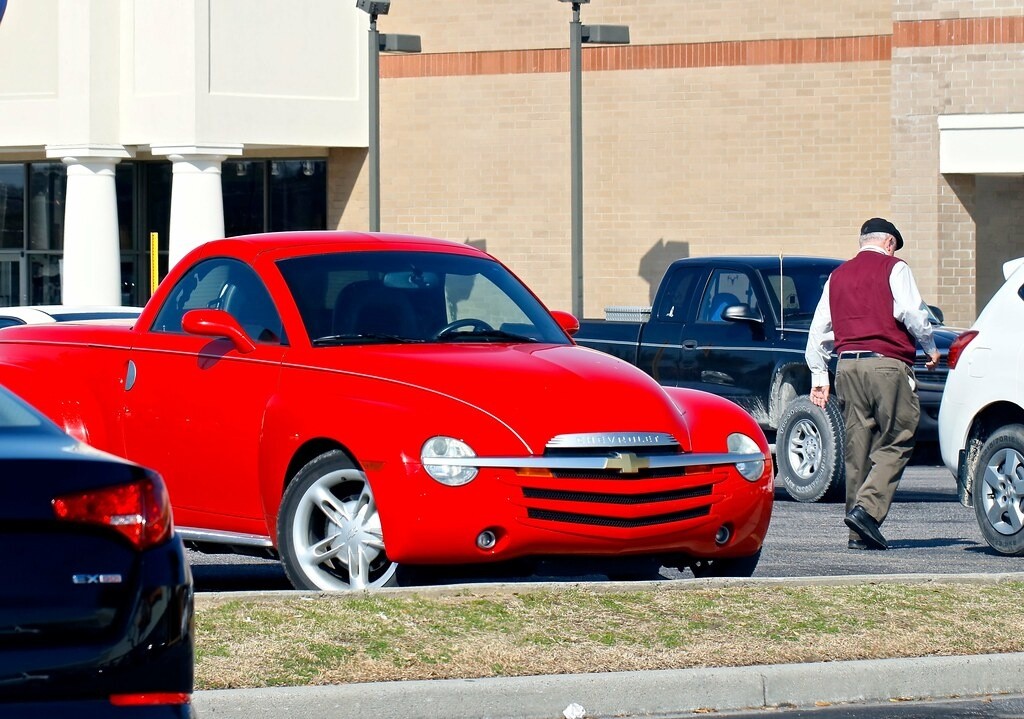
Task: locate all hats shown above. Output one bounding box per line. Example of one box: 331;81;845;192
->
861;218;903;251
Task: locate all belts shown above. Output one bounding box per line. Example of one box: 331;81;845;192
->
841;352;885;359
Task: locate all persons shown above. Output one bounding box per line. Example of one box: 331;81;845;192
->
805;217;939;550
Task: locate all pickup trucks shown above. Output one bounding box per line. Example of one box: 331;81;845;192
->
497;255;964;505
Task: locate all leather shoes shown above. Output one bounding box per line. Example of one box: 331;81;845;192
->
844;506;888;549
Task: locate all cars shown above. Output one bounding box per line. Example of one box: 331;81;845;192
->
1;303;143;330
0;382;196;719
938;256;1024;557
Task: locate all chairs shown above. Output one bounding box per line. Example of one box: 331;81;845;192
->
215;262;285;346
331;279;411;337
709;293;741;322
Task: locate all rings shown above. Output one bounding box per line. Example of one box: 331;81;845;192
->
932;360;936;364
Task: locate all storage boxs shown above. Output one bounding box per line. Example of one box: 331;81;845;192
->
604;305;652;322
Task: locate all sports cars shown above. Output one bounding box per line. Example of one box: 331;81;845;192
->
0;230;775;591
